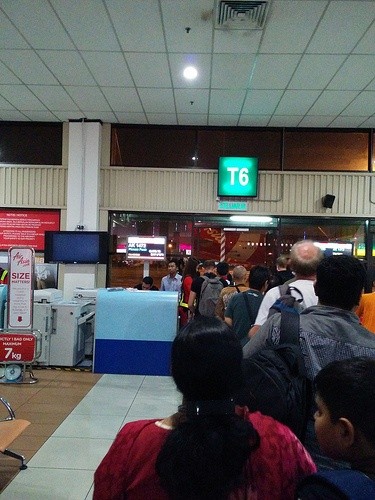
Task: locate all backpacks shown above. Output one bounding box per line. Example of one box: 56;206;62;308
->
267;285;306;319
198;275;224;318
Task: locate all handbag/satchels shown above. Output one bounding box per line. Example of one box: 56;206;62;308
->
232;306;308;448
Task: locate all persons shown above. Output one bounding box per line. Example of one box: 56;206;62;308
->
181;257;203;304
215;266;249;320
249;240;324;337
352;256;375;334
294;355;375;500
272;257;294;287
93;315;316;500
134;277;159;291
160;261;183;302
225;264;271;347
217;262;235;287
189;260;228;319
242;255;375;471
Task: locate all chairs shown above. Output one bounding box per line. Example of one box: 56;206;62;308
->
0;396;32;469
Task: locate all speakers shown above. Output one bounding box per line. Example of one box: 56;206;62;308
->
322;194;335;208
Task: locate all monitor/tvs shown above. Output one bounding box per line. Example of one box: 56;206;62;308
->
44;232;109;264
126;235;166;261
312;242;354;257
